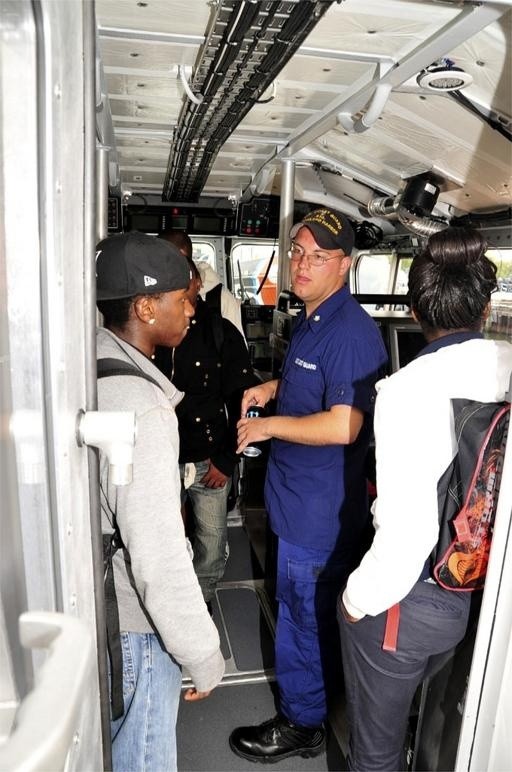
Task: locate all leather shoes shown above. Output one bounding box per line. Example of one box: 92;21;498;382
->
230;714;326;763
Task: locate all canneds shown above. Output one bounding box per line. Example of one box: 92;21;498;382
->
241;406;264;459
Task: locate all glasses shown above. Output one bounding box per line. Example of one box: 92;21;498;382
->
287;248;344;266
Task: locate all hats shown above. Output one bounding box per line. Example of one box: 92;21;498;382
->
289;207;355;256
96;229;190;301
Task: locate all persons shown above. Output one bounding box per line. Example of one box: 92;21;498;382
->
154;256;254;621
334;224;512;772
229;206;388;761
158;226;250;566
95;233;227;772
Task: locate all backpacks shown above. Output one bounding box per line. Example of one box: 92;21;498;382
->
428;399;511;591
